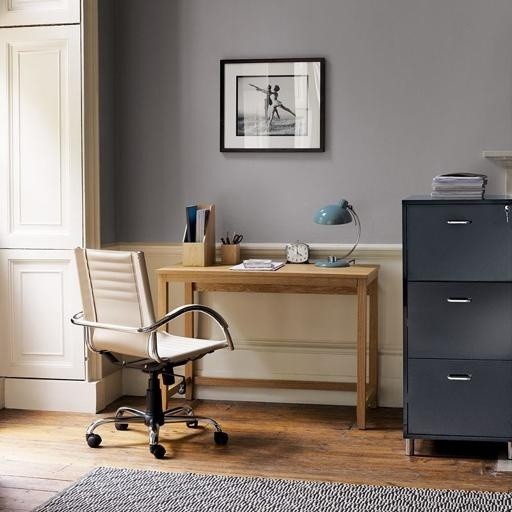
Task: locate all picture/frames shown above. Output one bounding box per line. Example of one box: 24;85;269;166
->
218;56;325;153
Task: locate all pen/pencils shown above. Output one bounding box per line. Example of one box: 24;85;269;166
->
220;232;241;244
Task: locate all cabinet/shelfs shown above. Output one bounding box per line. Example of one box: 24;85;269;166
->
1;1;124;414
399;195;512;460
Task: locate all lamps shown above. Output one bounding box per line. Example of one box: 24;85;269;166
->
313;198;362;267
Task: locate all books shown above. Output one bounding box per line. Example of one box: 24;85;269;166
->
184;204;209;242
242;258;274;268
229;259;285;272
428;172;489;200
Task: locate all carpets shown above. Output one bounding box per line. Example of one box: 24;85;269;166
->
29;465;511;512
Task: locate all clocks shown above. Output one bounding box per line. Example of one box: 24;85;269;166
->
284;239;312;265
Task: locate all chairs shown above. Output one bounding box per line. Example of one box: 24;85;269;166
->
70;246;236;460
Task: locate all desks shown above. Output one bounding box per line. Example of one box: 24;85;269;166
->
155;264;380;430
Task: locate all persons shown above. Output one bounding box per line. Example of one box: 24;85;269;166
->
254;85;296;134
247;82;272;121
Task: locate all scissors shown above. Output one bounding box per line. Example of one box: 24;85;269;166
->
233;234;243;244
